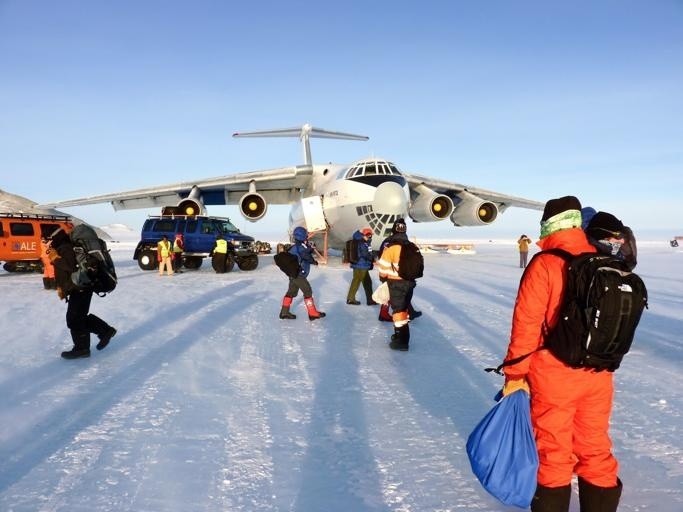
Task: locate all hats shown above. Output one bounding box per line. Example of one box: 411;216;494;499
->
361;228;374;236
541;197;581;224
390;217;406;233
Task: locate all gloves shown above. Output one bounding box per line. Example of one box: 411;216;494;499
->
157;255;162;262
502;371;530;398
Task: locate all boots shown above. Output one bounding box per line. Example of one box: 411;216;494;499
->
378;303;393;322
407;303;422;323
279;295;296;320
303;296;326;321
389;325;410;352
42;276;49;289
48;277;56;289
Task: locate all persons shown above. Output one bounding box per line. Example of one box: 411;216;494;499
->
210;235;227;274
517;235;531;268
41;222;116;360
277;226;326;320
156;235;175;276
373;236;422;322
498;192;623;512
345;227;380;306
378;217;415;352
170;232;185;273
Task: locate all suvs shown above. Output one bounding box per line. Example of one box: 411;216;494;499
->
0;213;82;273
136;213;257;275
254;240;272;255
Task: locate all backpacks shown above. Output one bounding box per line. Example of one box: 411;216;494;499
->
344;238;367;264
68;223;117;298
273;251;302;279
533;245;650;373
387;239;424;280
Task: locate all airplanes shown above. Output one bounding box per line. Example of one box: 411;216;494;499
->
37;124;545;262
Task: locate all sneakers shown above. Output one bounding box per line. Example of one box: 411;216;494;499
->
60;347;91;360
96;325;116;350
346;298;360;305
366;298;376;306
